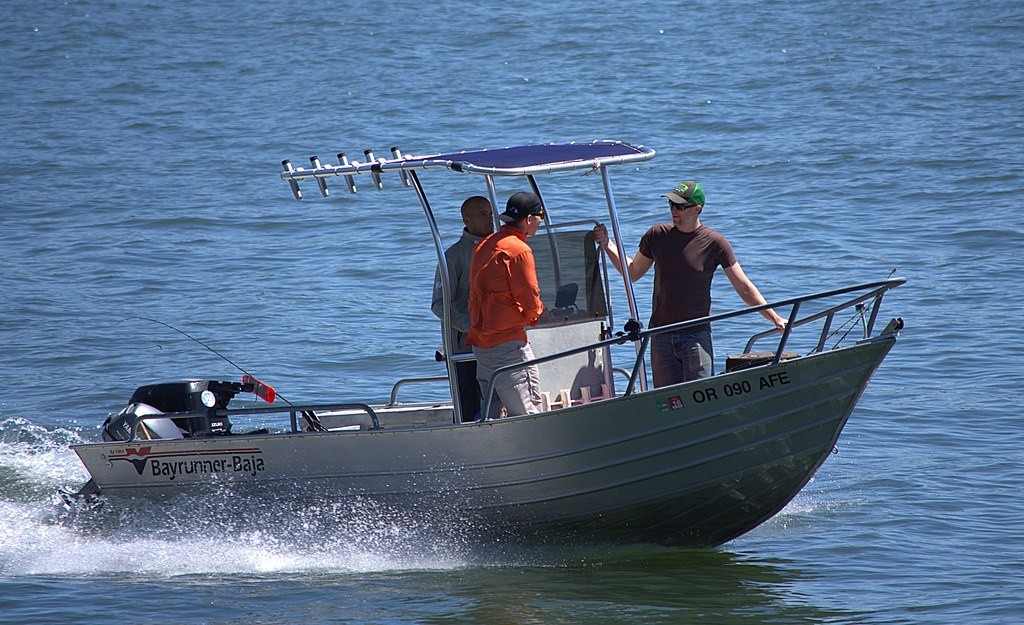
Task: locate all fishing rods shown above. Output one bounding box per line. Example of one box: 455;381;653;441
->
117;314;331;434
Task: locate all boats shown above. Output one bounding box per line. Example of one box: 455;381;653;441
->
66;138;908;548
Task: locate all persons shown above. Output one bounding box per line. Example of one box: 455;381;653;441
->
593;181;791;388
431;196;494;423
467;192;543;419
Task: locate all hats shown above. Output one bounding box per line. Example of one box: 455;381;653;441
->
661;180;705;206
495;191;542;222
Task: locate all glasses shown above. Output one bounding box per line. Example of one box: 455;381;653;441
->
531;210;545;220
669;199;698;211
